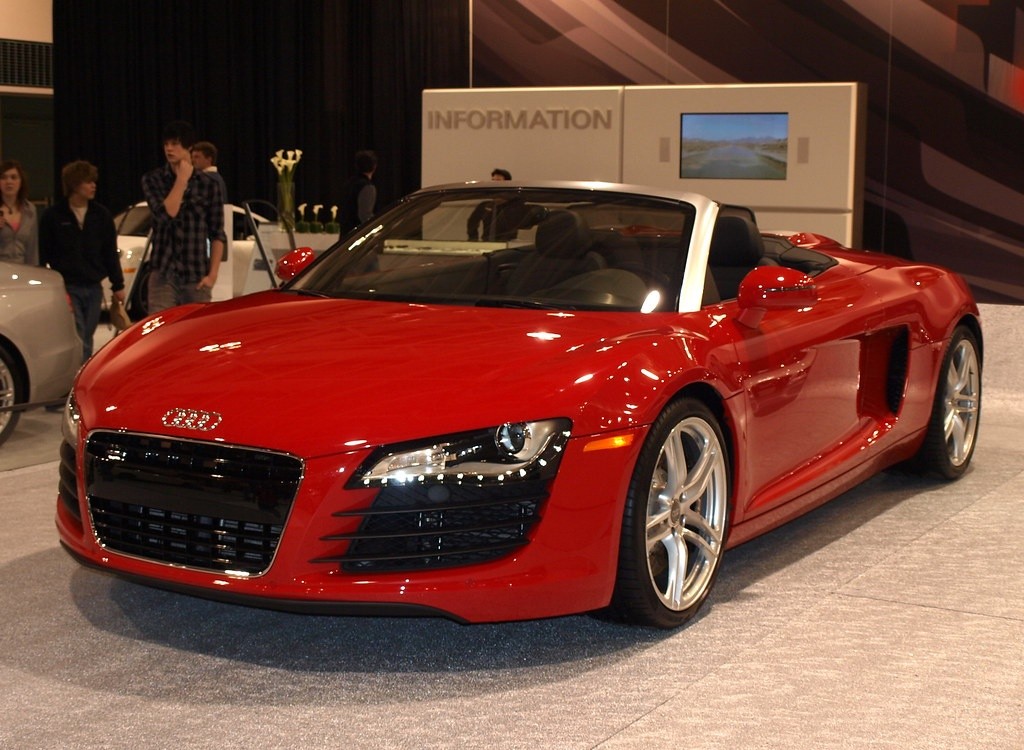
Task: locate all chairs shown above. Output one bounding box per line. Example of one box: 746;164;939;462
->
677;216;778;300
502;210;607;298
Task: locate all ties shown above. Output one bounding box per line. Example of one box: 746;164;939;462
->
488;203;498;241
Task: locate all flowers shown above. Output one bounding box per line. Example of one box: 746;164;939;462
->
311;205;324;219
297;203;307;219
330;205;338;221
270;149;303;221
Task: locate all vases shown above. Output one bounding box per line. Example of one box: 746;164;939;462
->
310;219;324;233
295;220;309;233
274;182;296;232
325;221;339;233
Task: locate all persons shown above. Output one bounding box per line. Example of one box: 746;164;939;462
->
192;142;227;205
38;162;125;363
142;121;228;317
0;160;39;267
466;169;519;241
340;151;380;272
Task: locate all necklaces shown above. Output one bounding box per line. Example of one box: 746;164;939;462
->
4;202;15;214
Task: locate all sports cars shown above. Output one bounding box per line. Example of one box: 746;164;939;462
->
58;180;986;631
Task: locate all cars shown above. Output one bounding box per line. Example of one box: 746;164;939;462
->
95;200;277;309
0;263;85;447
